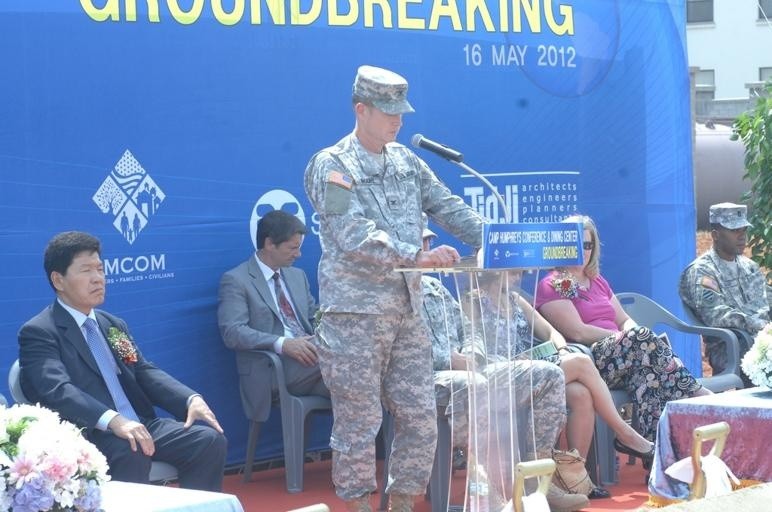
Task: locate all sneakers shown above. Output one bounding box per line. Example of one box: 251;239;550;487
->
543;479;593;511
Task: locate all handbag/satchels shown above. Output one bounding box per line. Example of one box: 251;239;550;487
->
549;448;595;498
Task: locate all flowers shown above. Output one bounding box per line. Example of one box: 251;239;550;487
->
1;402;109;512
741;322;772;390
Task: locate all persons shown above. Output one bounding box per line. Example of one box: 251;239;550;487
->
535;215;715;485
217;210;330;423
304;65;490;512
458;248;655;499
679;202;772;390
420;212;590;512
18;231;228;492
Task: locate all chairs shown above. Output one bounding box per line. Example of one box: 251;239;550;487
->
614;291;744;394
7;357;179;487
243;351;388;495
518;287;635;485
379;385;531;512
518;395;597;491
684;307;753;355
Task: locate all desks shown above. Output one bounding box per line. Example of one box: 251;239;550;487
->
106;479;242;510
648;385;772;506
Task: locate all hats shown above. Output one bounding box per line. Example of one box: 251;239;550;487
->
351;63;417;119
708;202;753;232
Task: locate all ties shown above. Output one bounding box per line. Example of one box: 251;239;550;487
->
272;272;311;338
83;317;144;425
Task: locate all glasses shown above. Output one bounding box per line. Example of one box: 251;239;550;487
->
583;242;594;250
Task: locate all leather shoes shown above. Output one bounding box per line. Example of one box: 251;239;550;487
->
612;433;658;461
588;487;610;498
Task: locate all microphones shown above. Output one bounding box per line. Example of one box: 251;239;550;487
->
410;132;465;168
